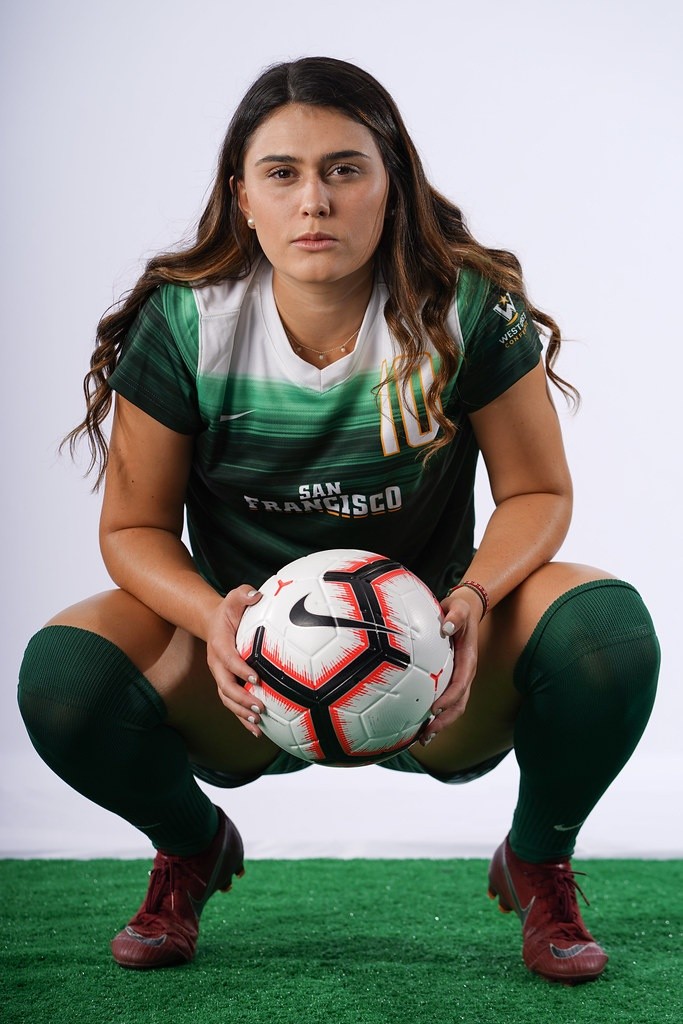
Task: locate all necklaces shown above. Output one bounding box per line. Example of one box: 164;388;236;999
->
285;327;359;359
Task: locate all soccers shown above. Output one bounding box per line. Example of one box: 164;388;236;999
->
230;546;459;770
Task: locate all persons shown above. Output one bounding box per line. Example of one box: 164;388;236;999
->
17;57;662;984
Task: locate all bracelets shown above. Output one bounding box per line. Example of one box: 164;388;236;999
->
445;580;491;624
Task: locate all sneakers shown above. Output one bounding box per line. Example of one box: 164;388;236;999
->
487;830;610;989
112;803;245;970
